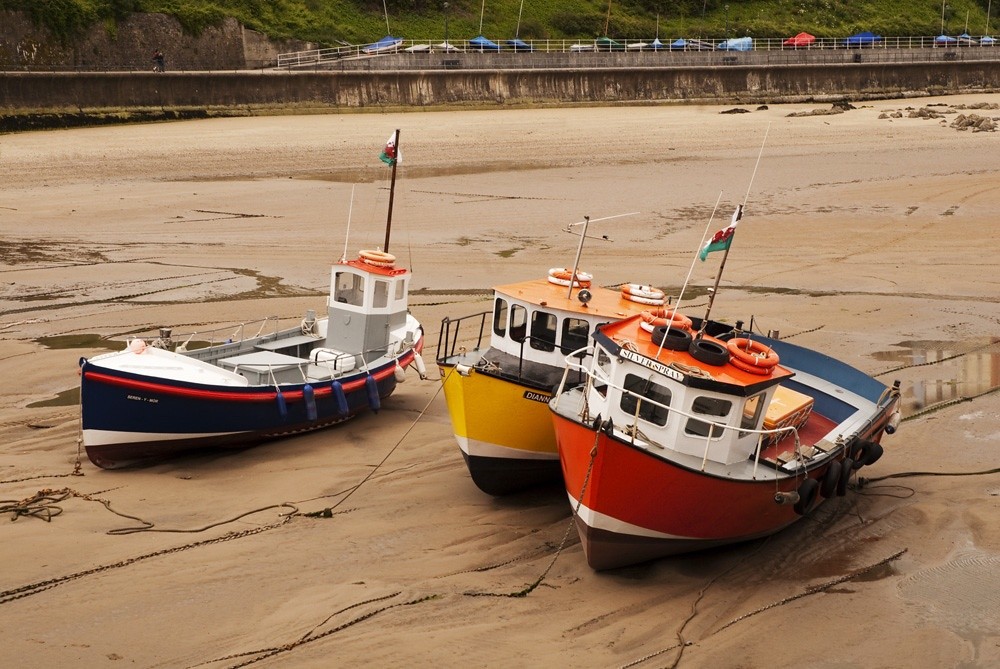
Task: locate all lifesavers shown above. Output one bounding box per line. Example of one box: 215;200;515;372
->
726;337;779;367
549;268;593;281
727;347;776;374
638;318;654;333
359;249;396;263
621;284;666;299
548;275;592;289
359;256;397;268
621;291;666;306
640;308;693;328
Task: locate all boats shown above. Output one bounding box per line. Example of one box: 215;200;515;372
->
73;126;427;472
551;122;902;571
434;213;669;501
